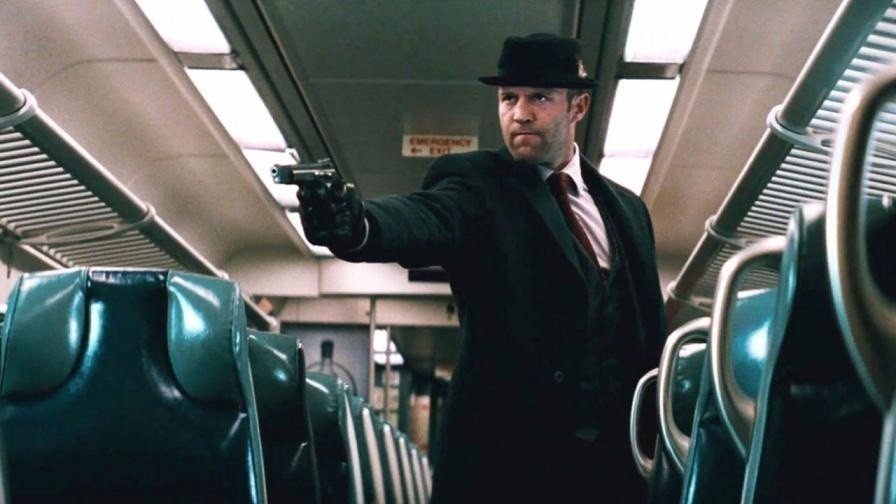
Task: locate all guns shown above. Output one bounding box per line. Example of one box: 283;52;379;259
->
270;157;341;190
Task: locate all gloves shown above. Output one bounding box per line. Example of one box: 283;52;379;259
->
297;179;366;252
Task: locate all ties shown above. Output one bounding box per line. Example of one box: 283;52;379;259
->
547;172;600;268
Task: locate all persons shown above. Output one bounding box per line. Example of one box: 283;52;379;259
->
298;32;662;503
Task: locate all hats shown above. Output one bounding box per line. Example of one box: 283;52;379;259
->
477;32;602;91
